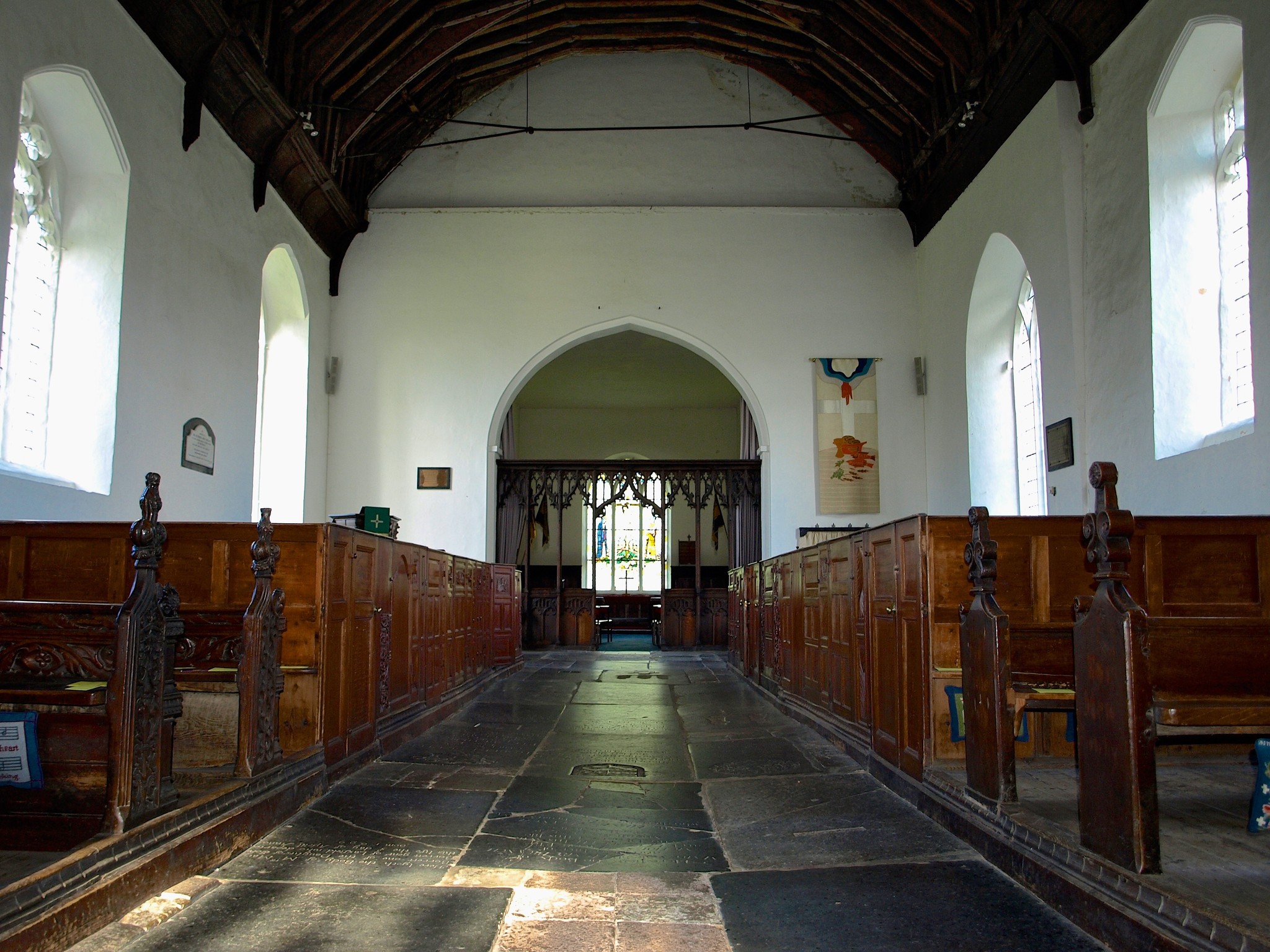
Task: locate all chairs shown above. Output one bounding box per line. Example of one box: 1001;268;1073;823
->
595;596;661;648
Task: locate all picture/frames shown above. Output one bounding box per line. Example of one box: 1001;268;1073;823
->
1046;417;1075;472
417;467;451;489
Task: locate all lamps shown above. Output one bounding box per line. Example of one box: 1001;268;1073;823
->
298;111;318;137
958;101;981;128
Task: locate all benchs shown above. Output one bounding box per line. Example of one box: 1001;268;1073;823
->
958;506;1076;806
0;472;184;835
1070;461;1269;875
171;508;285;775
